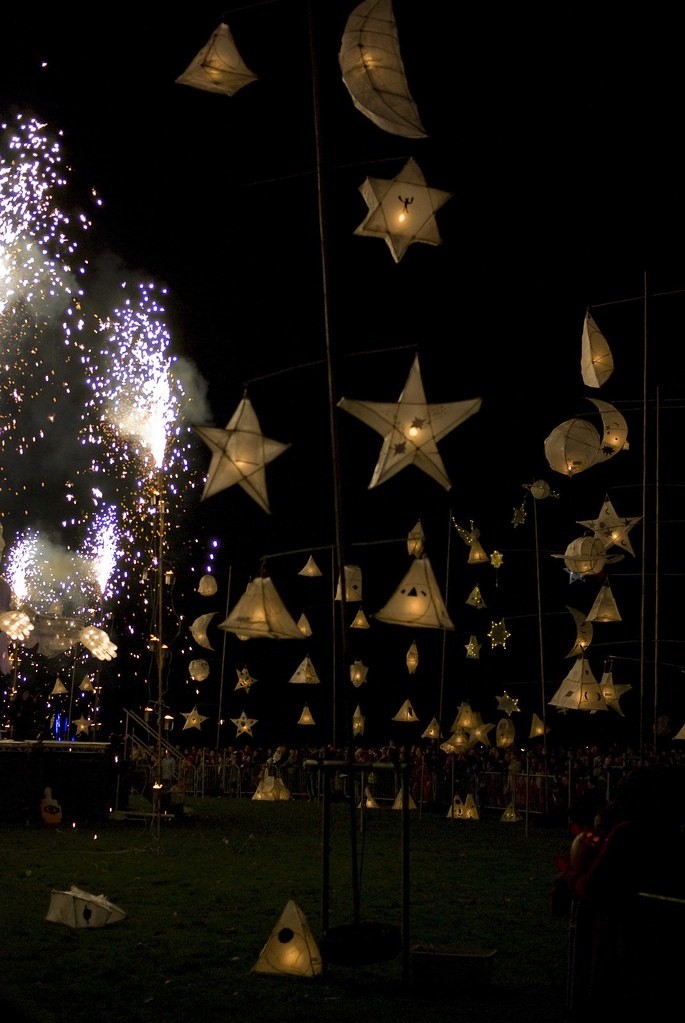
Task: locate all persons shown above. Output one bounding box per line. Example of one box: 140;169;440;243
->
109;730;685;930
